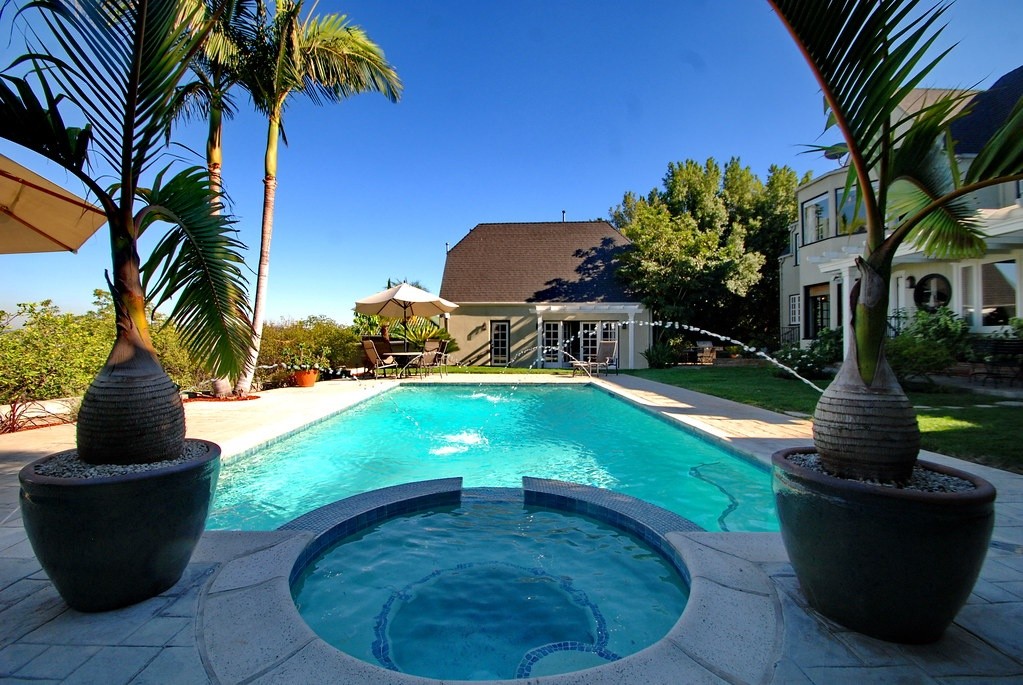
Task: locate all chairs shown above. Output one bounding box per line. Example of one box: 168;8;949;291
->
361;339;448;380
569;341;618;376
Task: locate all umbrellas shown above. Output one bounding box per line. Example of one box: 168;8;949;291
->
596;321;603;354
0;153;108;254
353;283;459;352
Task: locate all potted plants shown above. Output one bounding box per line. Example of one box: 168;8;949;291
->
280;342;332;388
0;0;259;613
769;0;1023;640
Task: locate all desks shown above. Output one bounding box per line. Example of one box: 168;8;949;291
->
383;352;422;377
